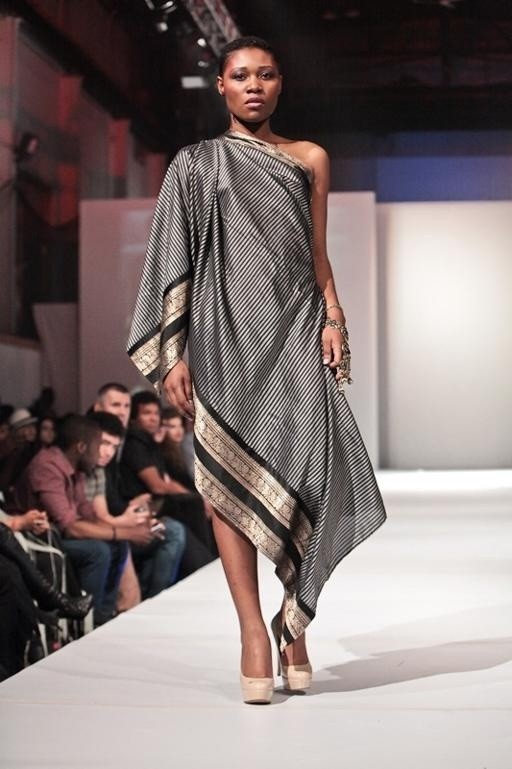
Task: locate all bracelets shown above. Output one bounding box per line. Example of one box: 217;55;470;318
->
326;303;343;312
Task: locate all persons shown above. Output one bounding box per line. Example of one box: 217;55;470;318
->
1;383;217;683
127;38;386;706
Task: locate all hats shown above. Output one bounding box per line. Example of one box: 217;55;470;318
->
10;408;38;433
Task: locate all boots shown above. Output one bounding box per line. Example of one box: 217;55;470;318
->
0;523;93;631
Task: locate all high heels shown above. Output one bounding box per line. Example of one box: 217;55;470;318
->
271;613;313;690
239;667;275;704
